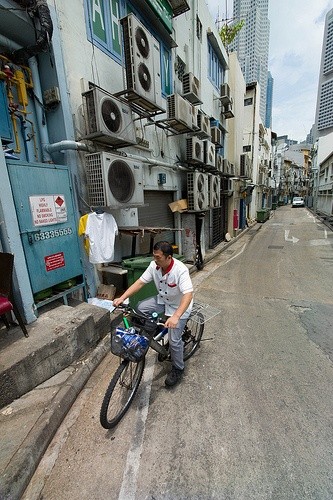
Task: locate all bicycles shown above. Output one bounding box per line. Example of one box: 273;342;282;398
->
99;302;204;430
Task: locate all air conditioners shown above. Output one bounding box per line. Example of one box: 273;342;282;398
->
260;173;271;187
81;88;137;149
167;72;234;209
86;152;144;208
120;14;162;112
240;154;251;177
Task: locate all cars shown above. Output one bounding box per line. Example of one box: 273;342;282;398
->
291;197;305;207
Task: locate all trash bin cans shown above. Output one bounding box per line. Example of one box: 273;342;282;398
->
123;253;187;321
257;210;267;222
279;201;282;205
272;203;277;210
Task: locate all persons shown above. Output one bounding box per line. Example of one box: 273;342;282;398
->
113;241;194;385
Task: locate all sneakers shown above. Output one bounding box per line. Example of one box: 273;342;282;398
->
165;366;185;385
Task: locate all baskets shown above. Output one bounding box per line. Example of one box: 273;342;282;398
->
110;317;156;363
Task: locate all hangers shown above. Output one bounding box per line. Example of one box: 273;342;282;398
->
90;206;105;213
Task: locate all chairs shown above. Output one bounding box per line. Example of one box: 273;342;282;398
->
0;252;29;337
